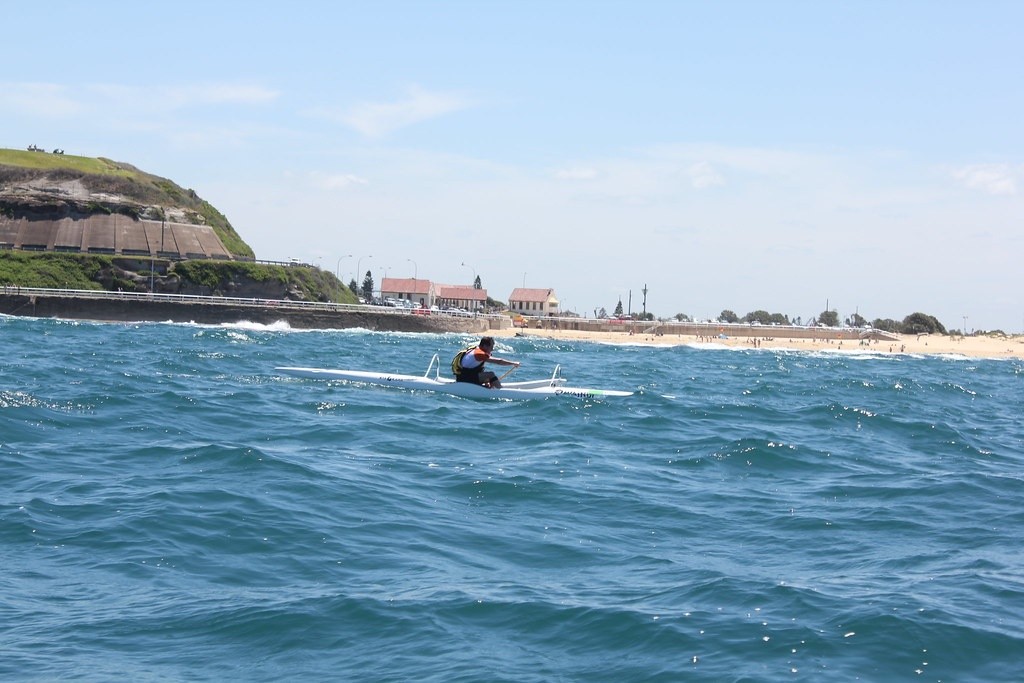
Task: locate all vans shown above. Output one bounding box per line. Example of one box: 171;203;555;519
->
291;258;302;266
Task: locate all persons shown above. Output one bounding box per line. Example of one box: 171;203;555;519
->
646;331;664;341
2;281;16;294
457;336;520;389
789;338;906;352
696;331;717;344
747;336;773;348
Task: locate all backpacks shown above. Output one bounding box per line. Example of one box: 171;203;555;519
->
450;346;484;375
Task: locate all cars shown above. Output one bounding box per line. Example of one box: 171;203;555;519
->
357;296;474;317
669;318;761;327
608;313;633;320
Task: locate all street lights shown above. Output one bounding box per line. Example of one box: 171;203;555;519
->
336;254;353;303
407;258;418;308
461;263;477;319
312;256;321;266
380;266;391;278
356;255;373;296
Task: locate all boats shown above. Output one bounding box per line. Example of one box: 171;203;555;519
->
274;354;633;401
521;318;528;327
516;328;529;337
536;319;543;328
